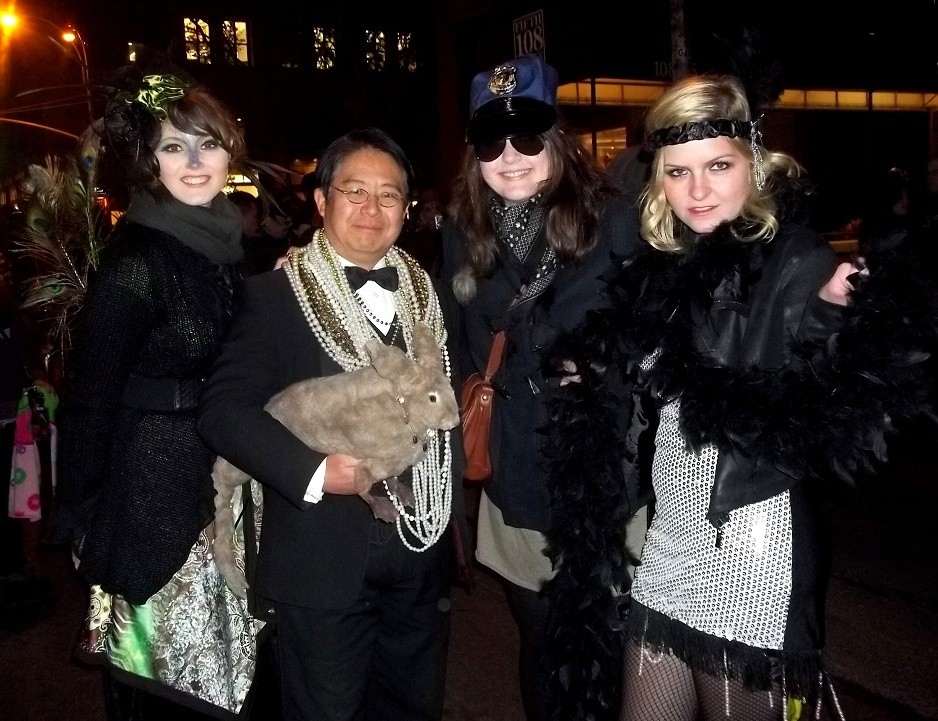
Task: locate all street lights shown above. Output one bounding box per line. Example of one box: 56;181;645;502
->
0;10;95;123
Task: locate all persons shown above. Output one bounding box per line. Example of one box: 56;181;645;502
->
194;126;462;721
540;73;938;721
226;151;467;288
76;71;259;721
405;54;614;721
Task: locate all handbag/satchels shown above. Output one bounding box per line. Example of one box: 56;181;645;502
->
457;374;495;480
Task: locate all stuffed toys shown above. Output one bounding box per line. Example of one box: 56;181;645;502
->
210;321;462;599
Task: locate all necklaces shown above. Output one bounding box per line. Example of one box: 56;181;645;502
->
281;222;468;554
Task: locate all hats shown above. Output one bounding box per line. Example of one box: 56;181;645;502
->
471;55;560;141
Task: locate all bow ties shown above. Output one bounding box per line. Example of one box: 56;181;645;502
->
345;266;397;291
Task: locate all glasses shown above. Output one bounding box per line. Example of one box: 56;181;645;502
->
474;133;544;163
327;185;407;208
